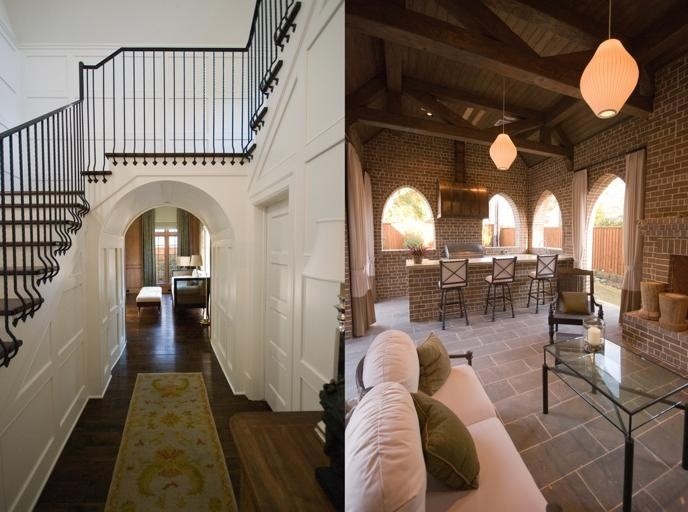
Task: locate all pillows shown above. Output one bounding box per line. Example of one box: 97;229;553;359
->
409;393;480;490
557;291;588;315
416;331;452;396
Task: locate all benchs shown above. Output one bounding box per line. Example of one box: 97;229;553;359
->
136;286;162;313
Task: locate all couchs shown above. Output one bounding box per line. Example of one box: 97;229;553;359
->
345;329;554;512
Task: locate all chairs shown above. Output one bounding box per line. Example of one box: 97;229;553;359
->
438;259;468;330
485;257;517;322
548;269;603;343
527;255;558;314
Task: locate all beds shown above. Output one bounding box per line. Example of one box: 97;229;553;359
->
542;335;688;511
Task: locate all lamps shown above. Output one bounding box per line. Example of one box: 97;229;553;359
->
176;256;190;276
580;1;640;119
489;78;518;170
190;254;203;276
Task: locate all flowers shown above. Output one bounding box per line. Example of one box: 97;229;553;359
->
404;240;431;256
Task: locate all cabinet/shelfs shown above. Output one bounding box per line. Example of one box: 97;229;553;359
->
172;270;210;317
231;412;344;512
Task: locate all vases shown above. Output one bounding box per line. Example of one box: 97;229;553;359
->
414;255;422;264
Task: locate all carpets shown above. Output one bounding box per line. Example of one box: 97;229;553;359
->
104;372;239;512
